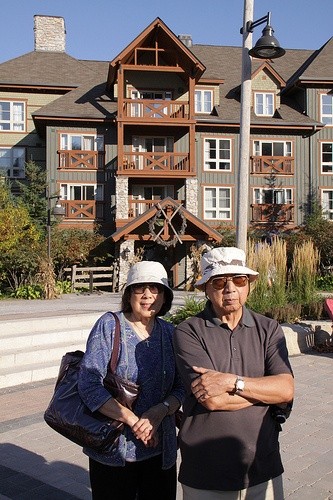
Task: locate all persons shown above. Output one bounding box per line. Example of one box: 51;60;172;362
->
78;260;187;500
171;246;295;500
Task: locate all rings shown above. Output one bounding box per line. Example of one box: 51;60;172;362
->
146;428;151;431
202;394;208;400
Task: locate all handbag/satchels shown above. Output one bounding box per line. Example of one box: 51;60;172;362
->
42;313;138;454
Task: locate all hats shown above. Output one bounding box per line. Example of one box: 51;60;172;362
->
123;261;174;317
194;247;258;292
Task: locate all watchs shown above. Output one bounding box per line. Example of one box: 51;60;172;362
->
161;400;170;413
232;375;246;396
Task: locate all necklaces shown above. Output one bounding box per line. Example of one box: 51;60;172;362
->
130;313;156;348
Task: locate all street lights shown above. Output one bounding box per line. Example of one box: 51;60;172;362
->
235;12;286;250
45;195;65;265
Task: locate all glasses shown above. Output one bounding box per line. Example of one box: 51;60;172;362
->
209;275;250;289
131;283;163;295
